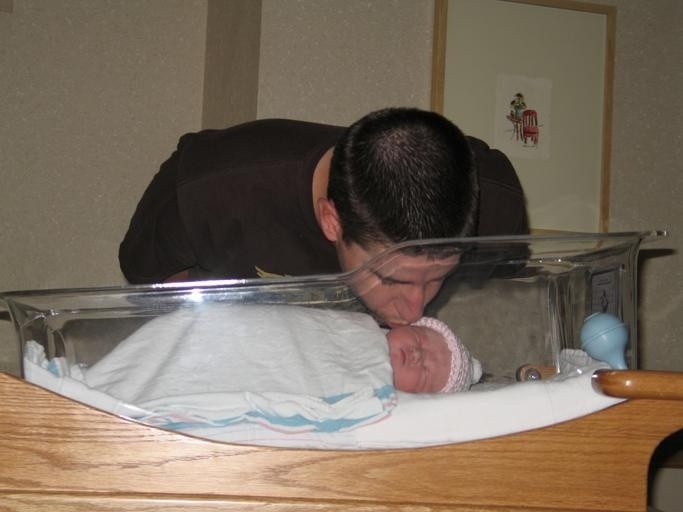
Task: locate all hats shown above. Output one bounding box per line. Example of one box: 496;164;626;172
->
409;316;483;397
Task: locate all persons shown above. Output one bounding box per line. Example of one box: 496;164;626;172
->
385;317;483;394
116;107;533;328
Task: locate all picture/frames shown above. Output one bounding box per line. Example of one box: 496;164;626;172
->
428;1;616;240
